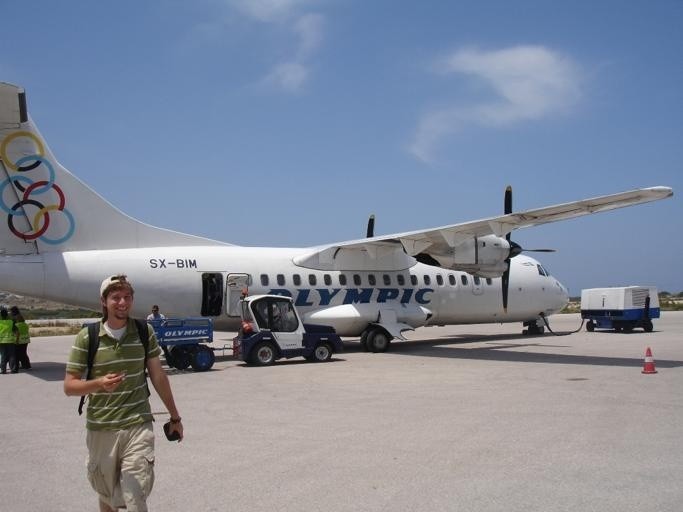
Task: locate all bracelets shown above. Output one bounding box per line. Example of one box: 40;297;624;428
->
169;415;181;423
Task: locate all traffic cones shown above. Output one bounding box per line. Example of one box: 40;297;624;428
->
641;345;657;374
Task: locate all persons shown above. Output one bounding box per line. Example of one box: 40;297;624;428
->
146;304;168;356
63;274;184;512
0;306;33;374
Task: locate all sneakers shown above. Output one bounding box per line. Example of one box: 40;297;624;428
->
0;366;32;373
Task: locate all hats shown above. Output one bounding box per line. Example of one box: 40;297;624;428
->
98;275;133;319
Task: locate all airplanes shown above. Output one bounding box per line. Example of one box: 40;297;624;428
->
0;81;673;352
